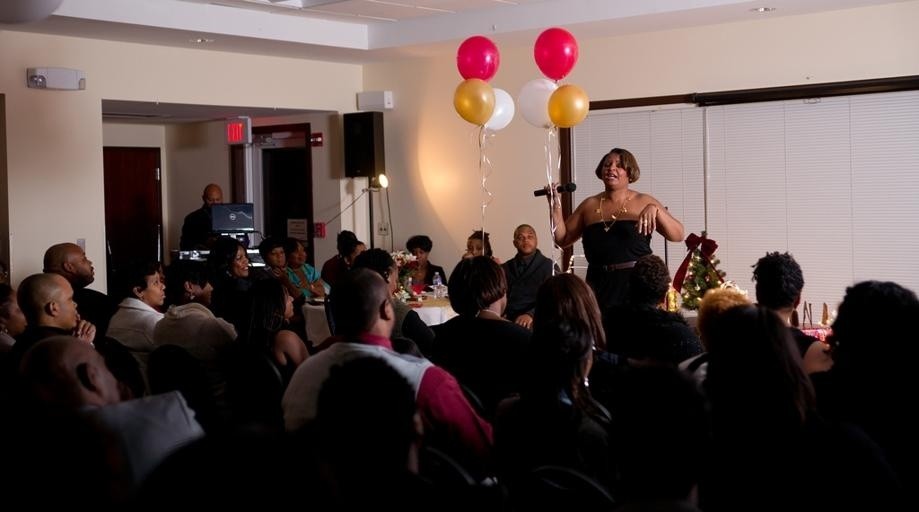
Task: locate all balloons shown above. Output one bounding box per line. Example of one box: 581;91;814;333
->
453;28;589;129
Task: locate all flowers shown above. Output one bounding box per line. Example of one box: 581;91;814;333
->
388;249;419;285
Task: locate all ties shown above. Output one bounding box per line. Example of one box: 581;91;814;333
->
518;260;524;274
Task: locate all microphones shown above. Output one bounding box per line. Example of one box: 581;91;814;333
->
534;183;576;196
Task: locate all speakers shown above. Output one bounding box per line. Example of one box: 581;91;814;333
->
343;111;385;176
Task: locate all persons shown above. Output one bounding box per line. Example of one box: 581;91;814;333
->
1;183;918;511
544;149;685;345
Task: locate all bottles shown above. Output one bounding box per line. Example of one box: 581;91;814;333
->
668;285;681;312
432;271;443;301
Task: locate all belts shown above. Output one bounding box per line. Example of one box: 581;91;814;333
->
602;261;638;272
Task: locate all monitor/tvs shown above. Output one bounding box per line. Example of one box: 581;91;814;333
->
211;203;255;231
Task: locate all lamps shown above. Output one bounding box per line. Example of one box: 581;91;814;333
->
26;66;88;91
343;174;389;212
